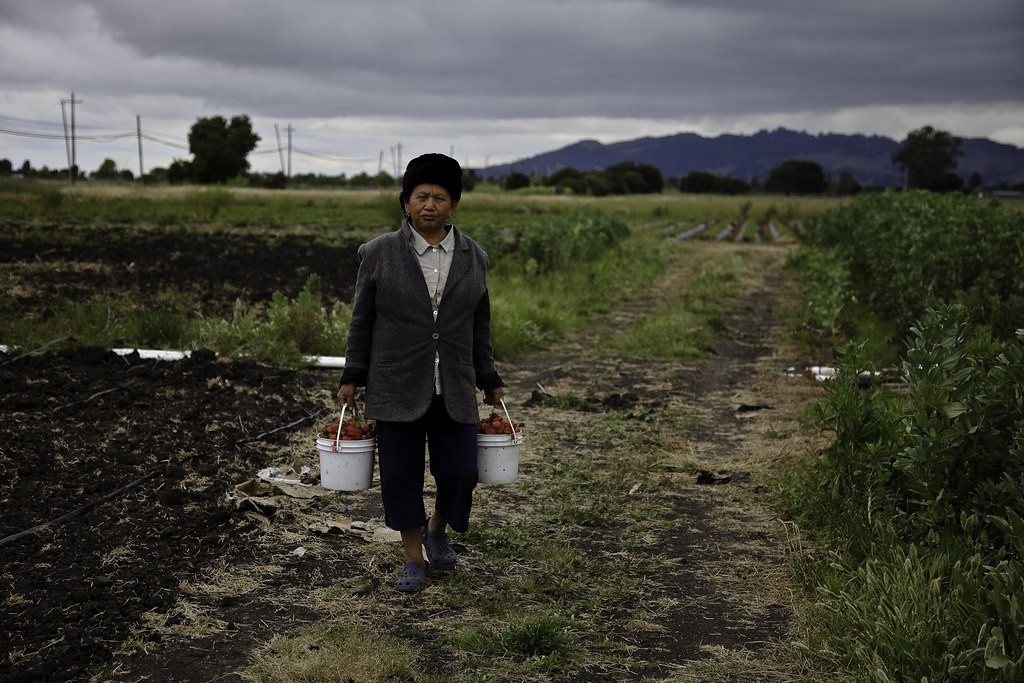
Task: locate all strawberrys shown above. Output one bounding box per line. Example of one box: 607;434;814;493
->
319;417;375;441
476;410;521;435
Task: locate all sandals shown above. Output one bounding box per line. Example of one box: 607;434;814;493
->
421;517;457;569
395;560;431;590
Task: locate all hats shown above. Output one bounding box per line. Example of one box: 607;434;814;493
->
403;154;463;205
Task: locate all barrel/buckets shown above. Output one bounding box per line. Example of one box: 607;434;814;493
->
314;402;376;491
475;398;523;485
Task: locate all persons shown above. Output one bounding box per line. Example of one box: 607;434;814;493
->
338;154;508;591
